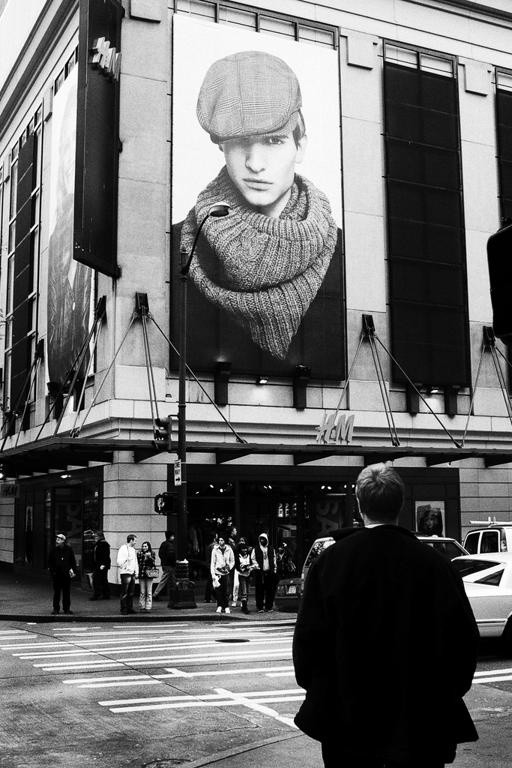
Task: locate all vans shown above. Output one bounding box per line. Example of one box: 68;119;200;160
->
300;534;470;602
462;521;512;554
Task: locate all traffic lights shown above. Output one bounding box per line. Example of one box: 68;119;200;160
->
153;416;172;454
153;493;174;514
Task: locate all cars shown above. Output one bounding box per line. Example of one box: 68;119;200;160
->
272;578;301;614
450;552;511;660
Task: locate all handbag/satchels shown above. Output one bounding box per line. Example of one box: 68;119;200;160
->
145;565;159;577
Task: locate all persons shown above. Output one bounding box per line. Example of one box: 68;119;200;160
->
43;524;308;609
166;49;351;386
288;462;489;768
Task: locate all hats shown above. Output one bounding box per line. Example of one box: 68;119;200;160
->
197;51;303;145
56;533;66;540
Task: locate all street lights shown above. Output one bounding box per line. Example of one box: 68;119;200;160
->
167;199;229;608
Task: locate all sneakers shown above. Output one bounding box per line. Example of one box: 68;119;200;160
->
224;607;230;613
216;605;222;612
